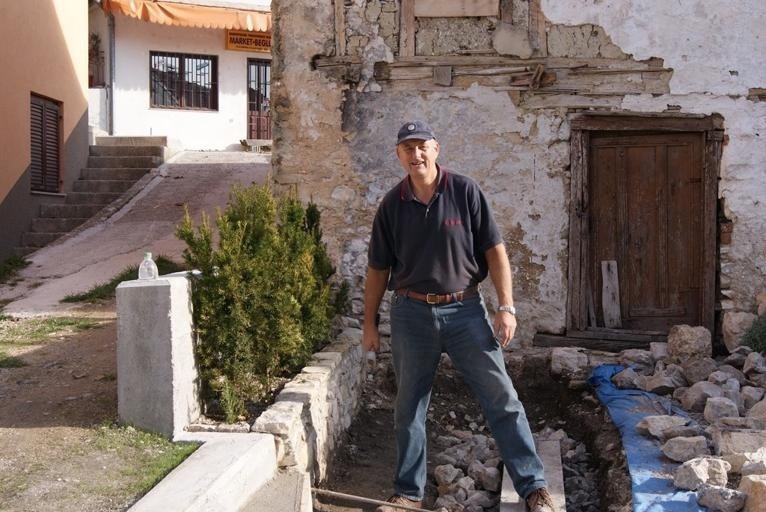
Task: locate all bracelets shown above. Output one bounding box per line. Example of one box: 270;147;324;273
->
497;305;517;317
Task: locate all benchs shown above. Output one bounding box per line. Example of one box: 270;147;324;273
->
240;139;273;153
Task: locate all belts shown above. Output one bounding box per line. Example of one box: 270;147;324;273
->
395;287;477;305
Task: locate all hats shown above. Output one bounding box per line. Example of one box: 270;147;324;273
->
396;120;436;146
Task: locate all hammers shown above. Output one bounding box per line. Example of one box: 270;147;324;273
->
365;314;377;370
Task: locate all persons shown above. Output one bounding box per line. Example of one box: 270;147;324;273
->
361;118;560;512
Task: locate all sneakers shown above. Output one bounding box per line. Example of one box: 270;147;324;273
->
524;487;556;512
374;494;422;512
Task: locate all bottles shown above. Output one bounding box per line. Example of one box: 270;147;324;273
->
365;312;381;386
137;252;158;279
